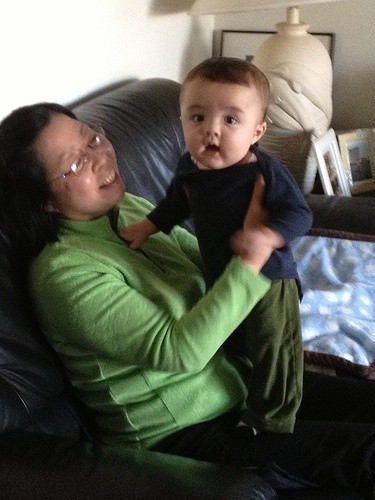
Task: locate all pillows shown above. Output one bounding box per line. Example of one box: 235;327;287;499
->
256;121;313;192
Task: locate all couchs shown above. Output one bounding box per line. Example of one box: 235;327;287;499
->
0;77;375;500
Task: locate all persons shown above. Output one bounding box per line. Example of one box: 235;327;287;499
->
0;102;375;500
118;56;314;466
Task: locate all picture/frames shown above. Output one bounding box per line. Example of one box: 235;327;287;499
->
219;30;336;69
312;128;375;196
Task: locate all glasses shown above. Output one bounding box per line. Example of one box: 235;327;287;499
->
47;123;105;185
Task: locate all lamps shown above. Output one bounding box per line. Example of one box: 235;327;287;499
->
187;0;341;194
